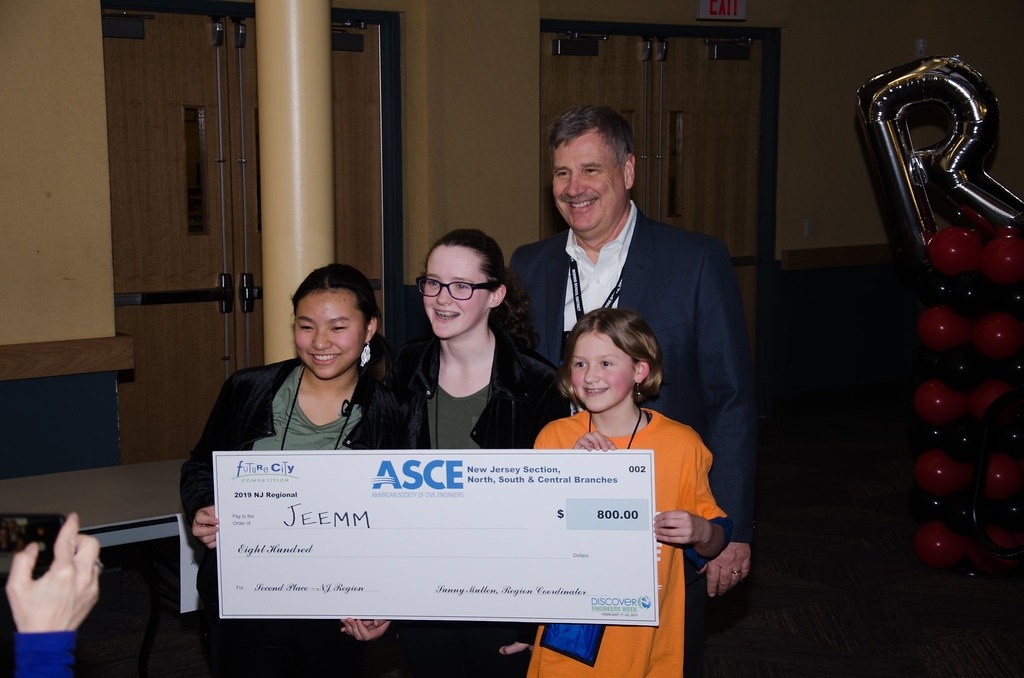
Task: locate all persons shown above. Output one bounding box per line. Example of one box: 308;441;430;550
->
179;265;406;677
5;511;101;678
505;103;758;677
386;228;567;678
525;308;733;678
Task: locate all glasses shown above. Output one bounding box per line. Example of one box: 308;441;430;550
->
416;276;499;300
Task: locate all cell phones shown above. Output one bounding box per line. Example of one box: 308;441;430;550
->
0;513;66;583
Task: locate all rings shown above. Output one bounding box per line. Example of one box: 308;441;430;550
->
732;570;741;574
94;560;104;575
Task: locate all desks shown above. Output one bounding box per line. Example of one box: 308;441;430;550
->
0;459;217;678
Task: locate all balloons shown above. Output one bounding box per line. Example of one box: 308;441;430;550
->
856;56;1024;269
913;229;1024;575
